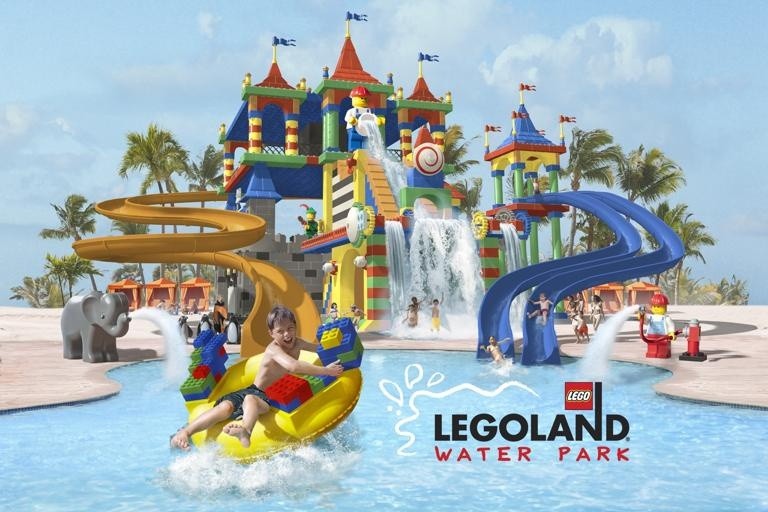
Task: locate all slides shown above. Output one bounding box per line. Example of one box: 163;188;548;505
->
477;192;685;366
72;190;323;357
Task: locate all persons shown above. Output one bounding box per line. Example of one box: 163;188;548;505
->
342;302;368;331
428;291;444;333
400;304;420;329
565;290;606;345
405;303;419;315
531;177;541;196
168;306;344;451
480;334;511;364
526;292;554;327
410;294;427;313
324;302;346;323
